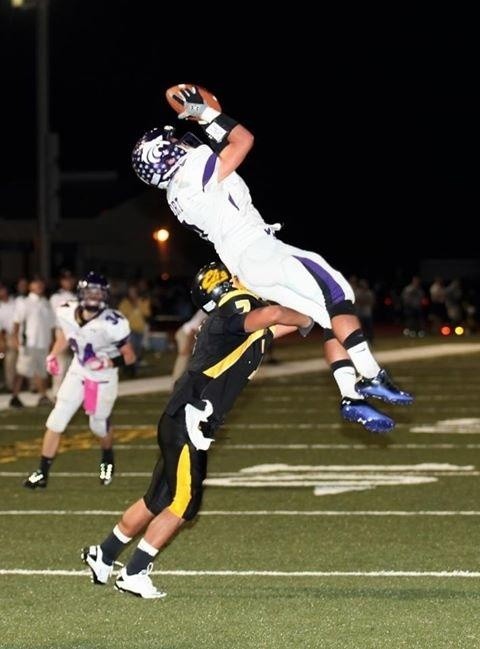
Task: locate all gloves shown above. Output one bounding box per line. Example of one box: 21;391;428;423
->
84;357;108;371
46;355;62;375
173;86;208;119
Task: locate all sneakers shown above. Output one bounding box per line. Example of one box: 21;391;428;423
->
23;469;47;489
114;566;167;599
340;397;395;433
99;455;114;485
81;545;115;585
355;368;414;406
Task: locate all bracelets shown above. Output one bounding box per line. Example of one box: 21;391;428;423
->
198;107;221;125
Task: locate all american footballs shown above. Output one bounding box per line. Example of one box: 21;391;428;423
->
165;83;222;122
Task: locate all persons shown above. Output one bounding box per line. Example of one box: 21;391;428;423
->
78;261;315;600
349;274;478;338
0;266;152;490
131;84;414;436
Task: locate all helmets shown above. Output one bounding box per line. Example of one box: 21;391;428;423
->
77;272;111;312
132;126;189;189
191;262;238;317
